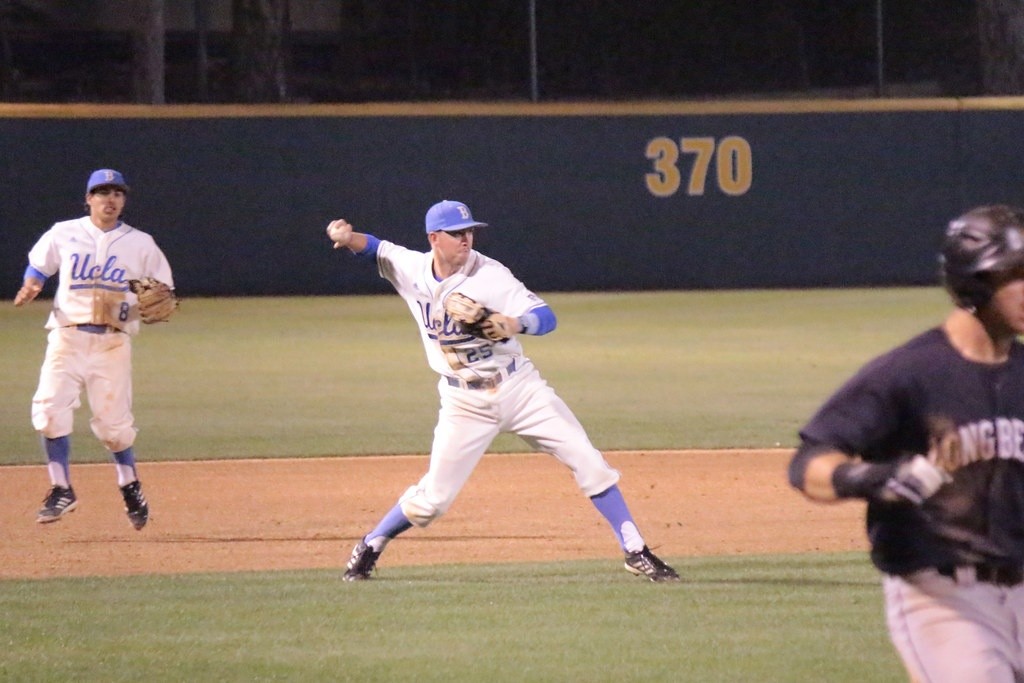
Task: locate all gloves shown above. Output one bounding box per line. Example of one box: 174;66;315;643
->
831;449;943;509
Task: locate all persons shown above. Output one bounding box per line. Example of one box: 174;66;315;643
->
786;204;1023;683
13;165;178;534
327;199;681;584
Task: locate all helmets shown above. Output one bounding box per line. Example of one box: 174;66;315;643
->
935;206;1024;309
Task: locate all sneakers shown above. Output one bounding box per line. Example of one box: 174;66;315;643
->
623;545;679;584
341;530;383;582
35;484;78;522
120;481;148;530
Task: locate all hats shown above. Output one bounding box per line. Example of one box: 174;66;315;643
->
86;170;130;196
425;199;489;233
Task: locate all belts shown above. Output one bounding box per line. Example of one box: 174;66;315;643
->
939;563;1024;585
446;360;516;390
65;324;121;335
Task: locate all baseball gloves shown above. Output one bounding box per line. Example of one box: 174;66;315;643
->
136;283;180;325
442;291;511;342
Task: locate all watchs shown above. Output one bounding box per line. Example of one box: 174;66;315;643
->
518;314;529;334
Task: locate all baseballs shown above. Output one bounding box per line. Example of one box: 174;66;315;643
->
328;227;347;242
